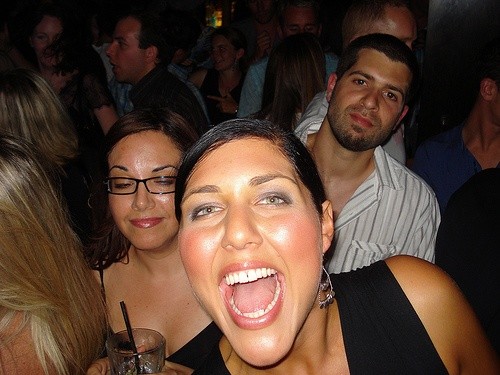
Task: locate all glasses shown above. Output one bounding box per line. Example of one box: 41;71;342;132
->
105;173;179;195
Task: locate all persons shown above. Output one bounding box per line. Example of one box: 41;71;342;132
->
237;0;322;119
229;0;283;66
410;33;500;215
0;130;111;375
295;33;440;274
84;107;224;375
14;0;87;97
247;33;327;134
163;118;500;375
188;27;249;127
0;69;79;168
295;1;417;153
94;10;211;137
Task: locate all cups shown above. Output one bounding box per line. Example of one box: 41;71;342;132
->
106;328;166;375
